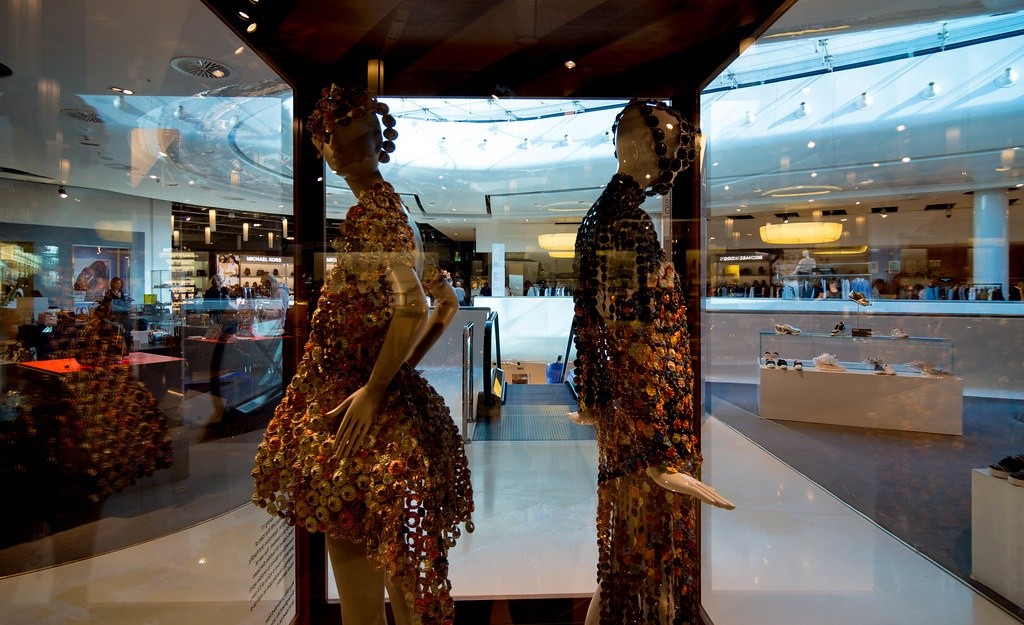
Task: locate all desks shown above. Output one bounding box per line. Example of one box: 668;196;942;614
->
188;334;296;408
970;467;1024;609
19;351;189;479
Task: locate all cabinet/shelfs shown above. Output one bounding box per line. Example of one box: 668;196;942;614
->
757;331;963;435
152;269;174;327
171;248;337;322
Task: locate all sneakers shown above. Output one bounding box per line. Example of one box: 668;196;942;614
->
830;321;844;336
765;360;776;368
775;324;801;335
892;327;908;338
1008;473;1024;486
816;359;845;371
874;364;896;375
849;290;872;306
921;363;946;375
777;360;788;368
793;360;803;370
987;454;1024;478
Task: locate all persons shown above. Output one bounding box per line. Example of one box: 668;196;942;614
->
260;272;289;328
102;277;133;356
249;83;473;624
572;99;735;624
792;249;817;281
218;255;239;286
454;281;465;306
203;275;228;324
480;282;491;296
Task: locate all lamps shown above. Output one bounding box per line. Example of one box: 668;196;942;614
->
52;182;953;261
